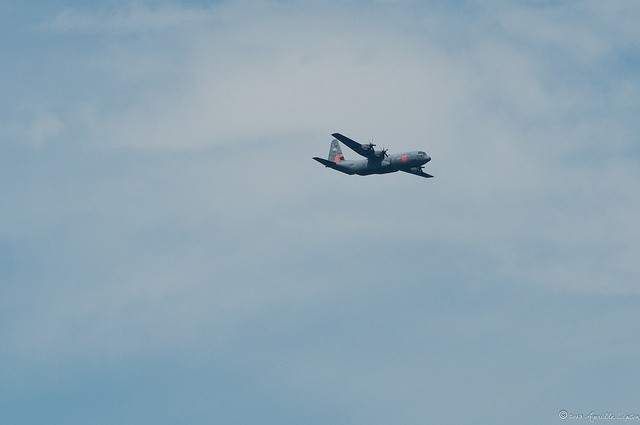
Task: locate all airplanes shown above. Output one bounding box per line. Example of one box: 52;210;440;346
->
312;132;434;178
